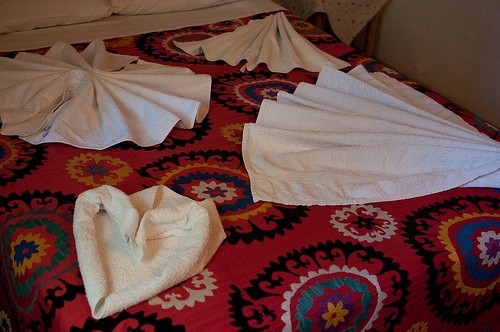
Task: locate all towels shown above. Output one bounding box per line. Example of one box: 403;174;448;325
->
242;64;500;206
73;184;227;319
0;40;212;150
173;12;351;74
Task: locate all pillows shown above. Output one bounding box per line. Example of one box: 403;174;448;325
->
109;0;239;16
0;0;113;35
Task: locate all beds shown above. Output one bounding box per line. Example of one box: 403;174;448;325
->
0;0;500;332
